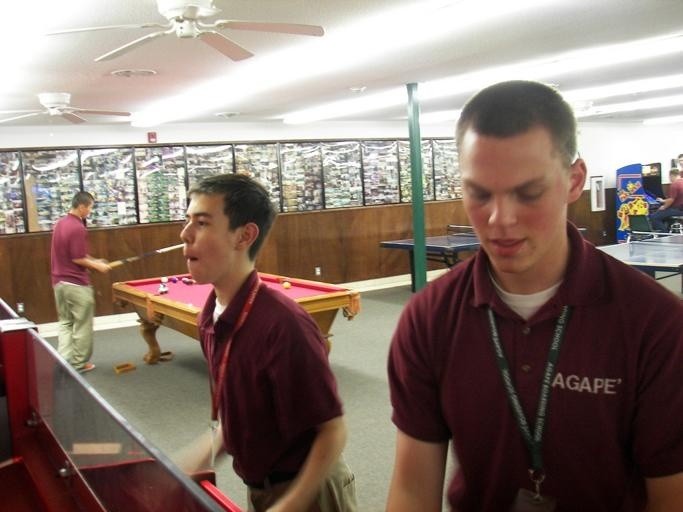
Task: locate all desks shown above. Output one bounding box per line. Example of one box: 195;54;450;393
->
590;225;683;292
377;223;483;292
110;265;361;373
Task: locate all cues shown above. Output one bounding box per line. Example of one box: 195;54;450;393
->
107;244;183;268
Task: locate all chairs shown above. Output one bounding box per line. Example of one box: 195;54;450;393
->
626;215;662;243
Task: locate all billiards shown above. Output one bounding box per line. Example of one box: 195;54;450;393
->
181;278;195;285
283;282;291;289
158;276;168;294
171;277;178;283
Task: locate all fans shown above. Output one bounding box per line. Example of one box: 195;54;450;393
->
0;93;132;126
48;1;329;66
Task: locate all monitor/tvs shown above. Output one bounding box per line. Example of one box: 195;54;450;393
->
645;192;656;201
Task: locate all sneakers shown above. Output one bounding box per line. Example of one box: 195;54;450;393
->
77;362;95;373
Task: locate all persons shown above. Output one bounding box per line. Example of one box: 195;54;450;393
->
651;153;683;237
386;81;683;512
50;192;112;373
180;174;356;512
